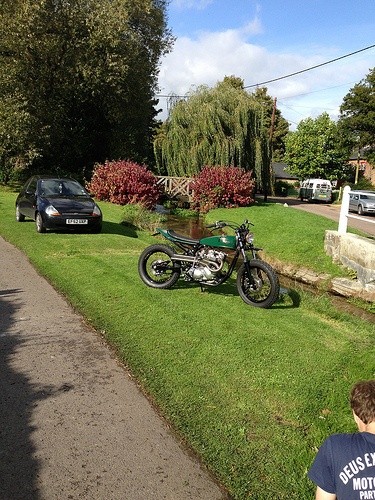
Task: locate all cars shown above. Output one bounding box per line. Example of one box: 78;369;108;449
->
350;190;375;195
14;174;103;233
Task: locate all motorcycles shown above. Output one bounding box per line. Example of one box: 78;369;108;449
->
138;220;280;308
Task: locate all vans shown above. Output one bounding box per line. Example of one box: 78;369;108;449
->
298;179;332;204
349;193;375;214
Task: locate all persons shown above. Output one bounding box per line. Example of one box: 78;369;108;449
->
307;380;375;500
